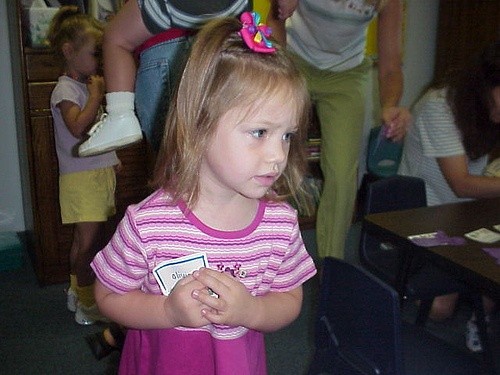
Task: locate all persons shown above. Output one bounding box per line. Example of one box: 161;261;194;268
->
397;42;500;356
89;11;317;375
49;0;412;325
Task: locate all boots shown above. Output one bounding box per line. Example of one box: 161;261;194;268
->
78;91;144;157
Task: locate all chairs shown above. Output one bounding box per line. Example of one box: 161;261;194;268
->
359;175;461;327
366;126;404;177
305;256;486;375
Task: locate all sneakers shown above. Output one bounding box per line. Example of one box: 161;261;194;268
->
67;288;112;325
465;313;489;352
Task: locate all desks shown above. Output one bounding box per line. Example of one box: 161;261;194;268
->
363;199;500;358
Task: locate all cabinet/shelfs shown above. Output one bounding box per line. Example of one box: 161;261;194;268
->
4;0;323;286
